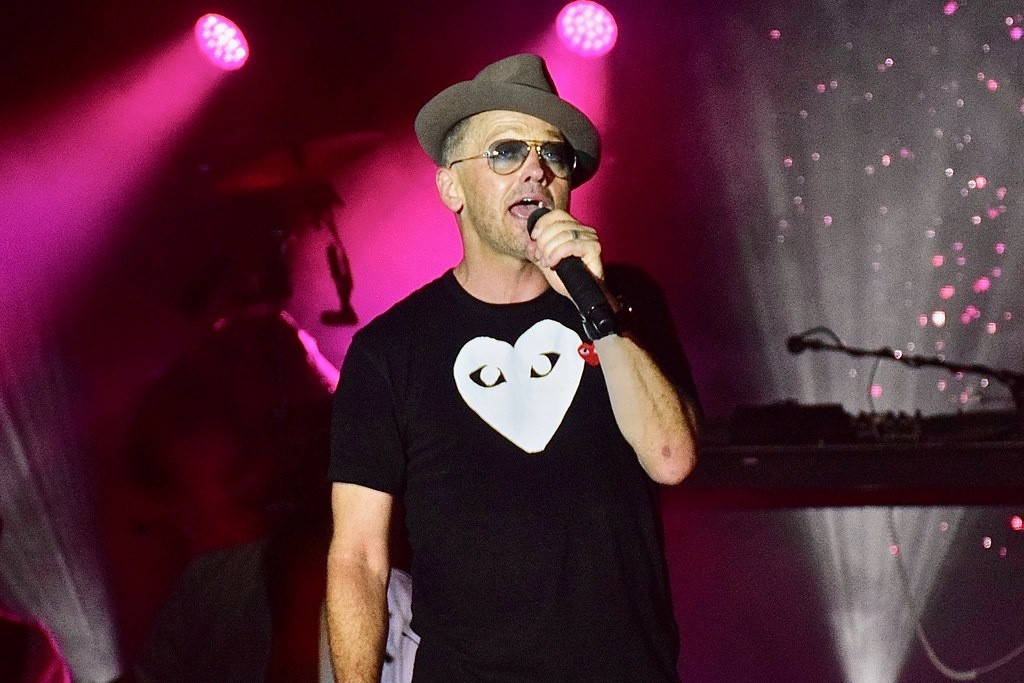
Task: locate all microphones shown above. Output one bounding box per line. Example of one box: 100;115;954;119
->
527;206;615;337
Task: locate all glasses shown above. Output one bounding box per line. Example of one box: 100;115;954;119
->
450;138;576;179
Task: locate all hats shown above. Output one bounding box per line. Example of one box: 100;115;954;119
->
414;54;602;191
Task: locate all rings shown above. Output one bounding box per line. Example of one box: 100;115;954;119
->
570;230;580;239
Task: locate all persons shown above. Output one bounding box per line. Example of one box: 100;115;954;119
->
319;50;705;683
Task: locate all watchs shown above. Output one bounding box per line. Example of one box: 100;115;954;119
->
583;301;635;340
